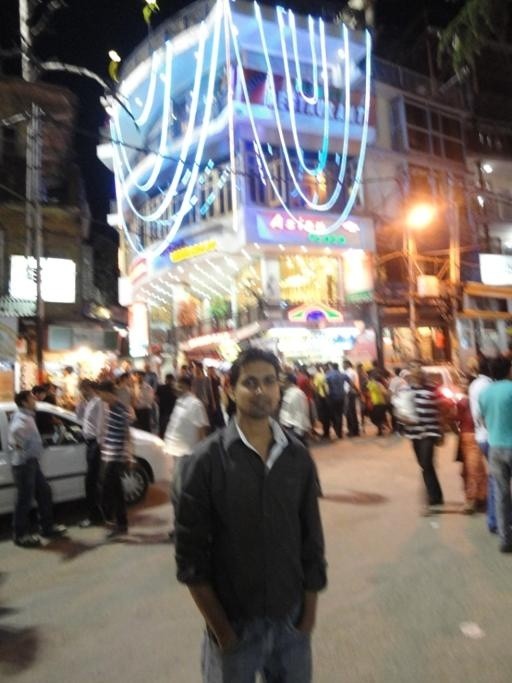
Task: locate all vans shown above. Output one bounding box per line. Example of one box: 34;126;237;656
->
394;362;469;435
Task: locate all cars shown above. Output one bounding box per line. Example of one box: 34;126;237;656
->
0;401;172;523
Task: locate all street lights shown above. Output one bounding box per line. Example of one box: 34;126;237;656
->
406;198;439;356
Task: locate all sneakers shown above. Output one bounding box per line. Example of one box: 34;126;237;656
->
13;517;126;548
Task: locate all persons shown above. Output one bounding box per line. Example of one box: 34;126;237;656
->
172;348;328;682
